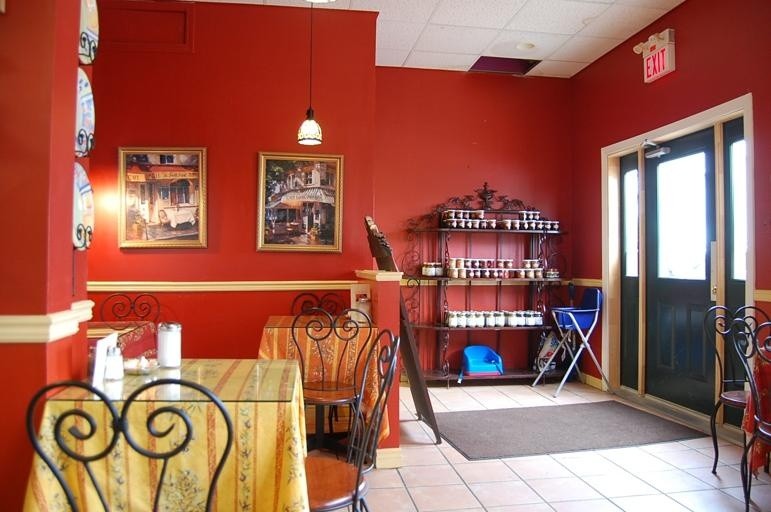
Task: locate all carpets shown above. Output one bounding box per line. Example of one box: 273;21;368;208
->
434;399;715;461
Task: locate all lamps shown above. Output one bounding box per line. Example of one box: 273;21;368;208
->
297;0;322;146
631;29;678;58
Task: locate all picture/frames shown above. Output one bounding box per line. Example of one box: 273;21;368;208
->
254;150;345;254
116;146;209;251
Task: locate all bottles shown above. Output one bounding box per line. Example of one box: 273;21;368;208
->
356;297;372;323
105;381;124;399
442;209;560;231
106;348;126;380
422;262;443;277
446;309;543;328
156;322;182;371
449;255;560;280
157;369;182;400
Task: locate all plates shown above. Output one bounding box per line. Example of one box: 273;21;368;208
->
125;366;154;374
69;0;102;251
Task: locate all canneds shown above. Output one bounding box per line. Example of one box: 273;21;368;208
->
444;310;543;328
447;258;559;278
422;262;443;277
443;209;560;230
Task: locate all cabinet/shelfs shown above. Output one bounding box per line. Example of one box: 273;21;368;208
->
397;185;568;387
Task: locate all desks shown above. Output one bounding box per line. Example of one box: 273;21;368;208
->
44;358;301;512
87;321;157;372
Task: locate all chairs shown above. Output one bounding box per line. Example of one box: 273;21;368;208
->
727;317;770;512
258;292;381;460
99;293;160;322
303;329;400;511
530;285;618;402
699;302;771;475
26;377;233;511
